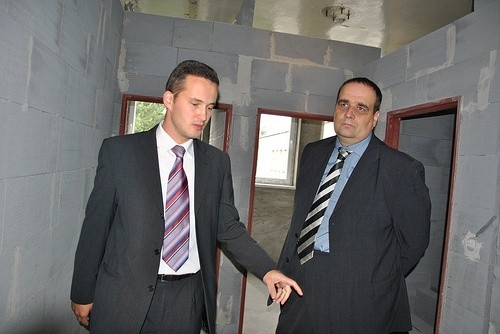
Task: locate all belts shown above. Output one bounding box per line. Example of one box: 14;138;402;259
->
157;269;202;280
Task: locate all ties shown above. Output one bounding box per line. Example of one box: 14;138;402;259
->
162;145;191;272
296;148;353;266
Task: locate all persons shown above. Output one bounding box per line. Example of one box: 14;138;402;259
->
267;77;432;334
68;59;303;334
